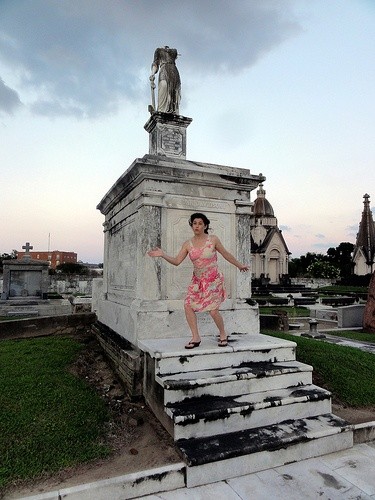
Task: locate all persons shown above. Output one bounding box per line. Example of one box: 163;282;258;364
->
147;213;249;349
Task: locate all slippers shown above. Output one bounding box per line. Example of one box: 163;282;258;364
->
218;335;228;346
184;340;201;349
